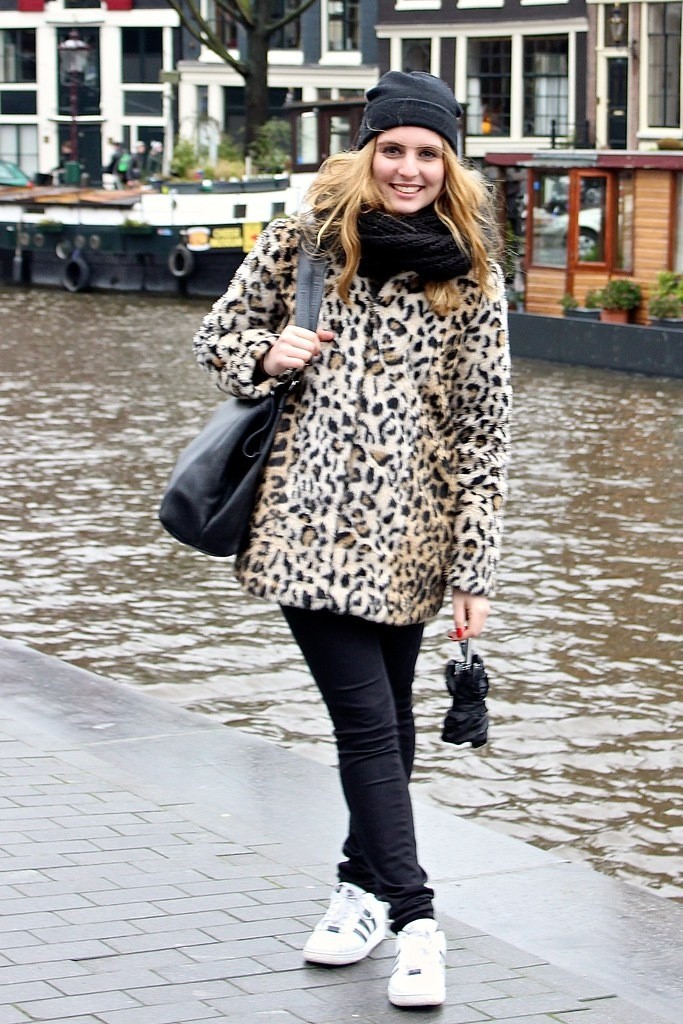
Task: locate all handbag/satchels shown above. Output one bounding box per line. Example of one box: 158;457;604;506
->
159;238;329;557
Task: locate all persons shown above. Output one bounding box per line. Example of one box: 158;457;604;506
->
47;138;163;189
188;68;511;1009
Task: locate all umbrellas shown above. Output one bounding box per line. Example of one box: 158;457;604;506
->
0;157;36;189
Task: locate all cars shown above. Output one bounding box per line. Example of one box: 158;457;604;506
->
0;161;35;187
543;195;624;263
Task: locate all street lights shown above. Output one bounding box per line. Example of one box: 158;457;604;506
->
56;33;91;182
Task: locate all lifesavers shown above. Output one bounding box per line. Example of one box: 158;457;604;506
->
168;246;192;275
59;258;90;293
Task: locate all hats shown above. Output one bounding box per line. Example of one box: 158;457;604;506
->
357;71;463;156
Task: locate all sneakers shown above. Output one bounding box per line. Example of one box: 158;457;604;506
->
388;918;446;1007
304;882;391;964
